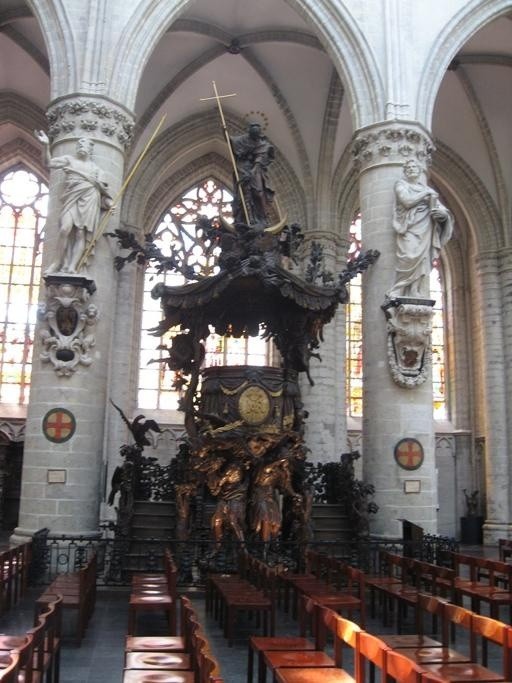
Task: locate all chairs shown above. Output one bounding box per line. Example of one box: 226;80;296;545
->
1;541;104;680
196;540;512;680
120;540;220;680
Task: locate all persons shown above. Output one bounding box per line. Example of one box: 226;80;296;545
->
220;121;282;234
34;129;114;273
383;159;455;301
